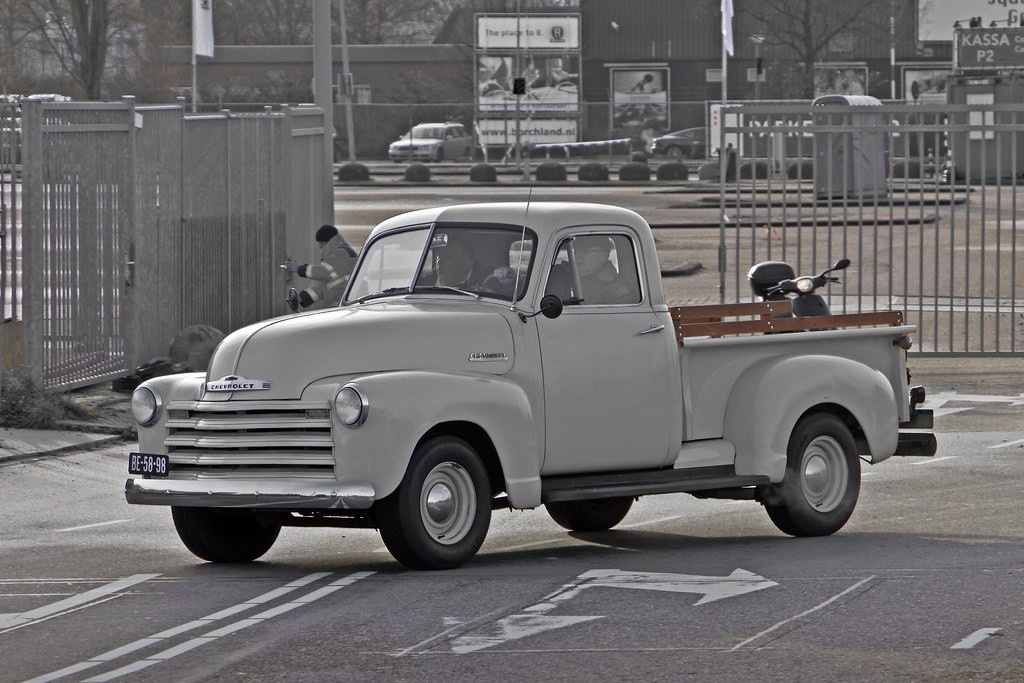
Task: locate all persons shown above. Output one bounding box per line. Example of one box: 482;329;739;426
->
284;224;369;308
416;233;496;293
493;235;637;305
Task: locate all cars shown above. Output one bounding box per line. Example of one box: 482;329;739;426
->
388;118;478;165
650;125;759;160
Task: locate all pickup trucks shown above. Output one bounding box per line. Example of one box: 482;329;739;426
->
121;197;940;572
